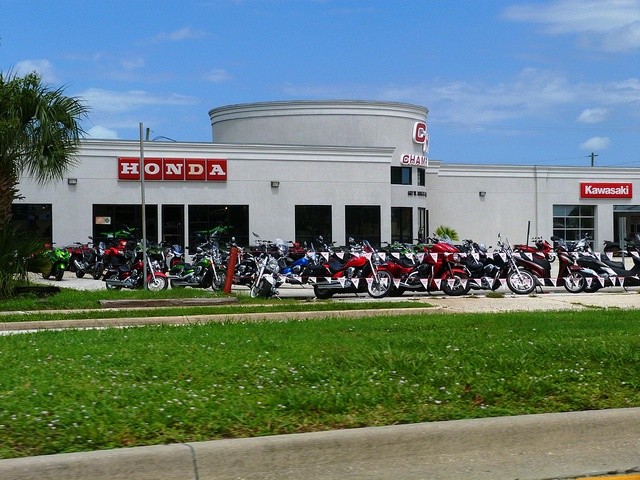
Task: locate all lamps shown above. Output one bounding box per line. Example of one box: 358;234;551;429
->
67;178;77;184
479;191;486;196
271;181;279;187
